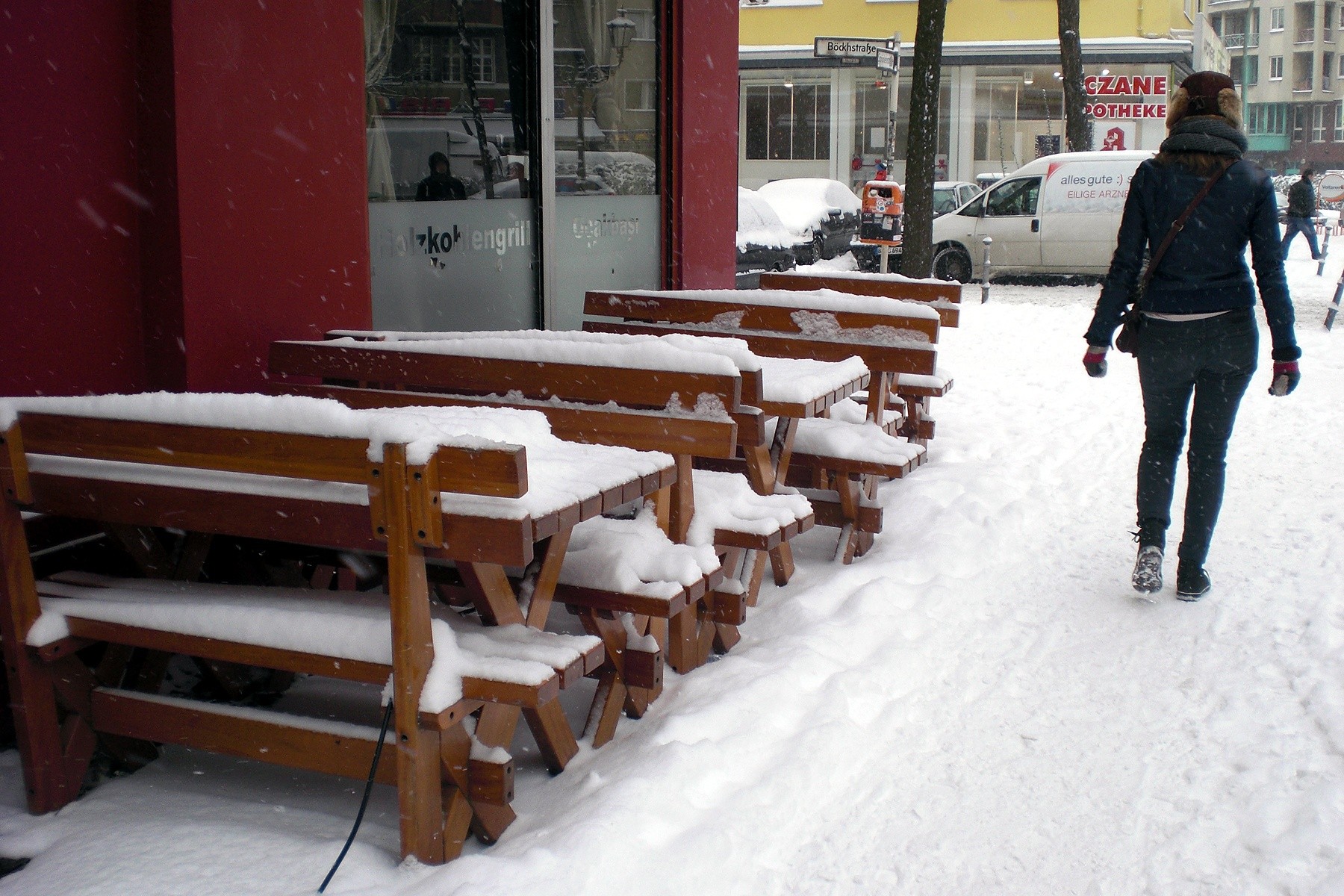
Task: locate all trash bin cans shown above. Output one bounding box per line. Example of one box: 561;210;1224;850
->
860;181;902;240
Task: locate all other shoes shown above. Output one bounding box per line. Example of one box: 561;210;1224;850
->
1315;252;1329;260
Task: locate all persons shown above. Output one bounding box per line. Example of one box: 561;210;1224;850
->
507;162;528;198
1282;168;1322;260
416;152;466;201
1083;71;1302;600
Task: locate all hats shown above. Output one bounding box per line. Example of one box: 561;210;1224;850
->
429;151;450;172
874;163;888;170
1165;70;1243;129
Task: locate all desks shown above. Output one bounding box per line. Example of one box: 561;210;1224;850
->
19;461;677;863
723;370;872;609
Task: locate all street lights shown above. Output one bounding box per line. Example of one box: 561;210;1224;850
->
553;0;636;176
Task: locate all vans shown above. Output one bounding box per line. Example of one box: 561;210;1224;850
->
931;149;1163;285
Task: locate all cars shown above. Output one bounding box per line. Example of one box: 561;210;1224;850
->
734;179;862;276
899;181;988;216
1274;191;1290;224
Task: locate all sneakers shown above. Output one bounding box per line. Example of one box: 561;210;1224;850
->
1128;524;1165;593
1175;568;1212;601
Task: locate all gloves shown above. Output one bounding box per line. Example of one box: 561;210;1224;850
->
1267;359;1301;396
1082;345;1108;378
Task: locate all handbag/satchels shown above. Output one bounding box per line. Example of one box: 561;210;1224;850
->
1115;307;1142;358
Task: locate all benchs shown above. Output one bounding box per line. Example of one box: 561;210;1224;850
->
0;274;964;865
